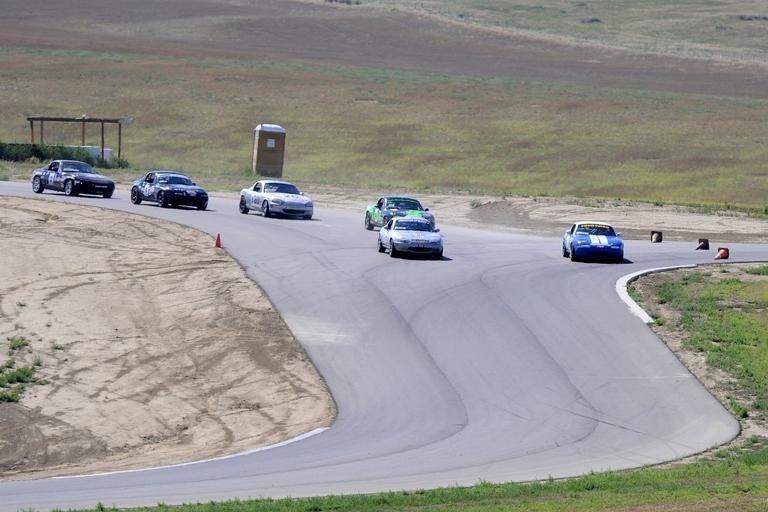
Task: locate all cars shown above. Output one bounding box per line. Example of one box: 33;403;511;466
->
364;196;443;258
130;171;208;211
31;159;115;198
562;220;624;263
239;180;313;219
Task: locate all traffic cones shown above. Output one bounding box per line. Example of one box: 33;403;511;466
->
715;247;730;260
213;233;221;248
651;231;661;242
695;239;709;251
